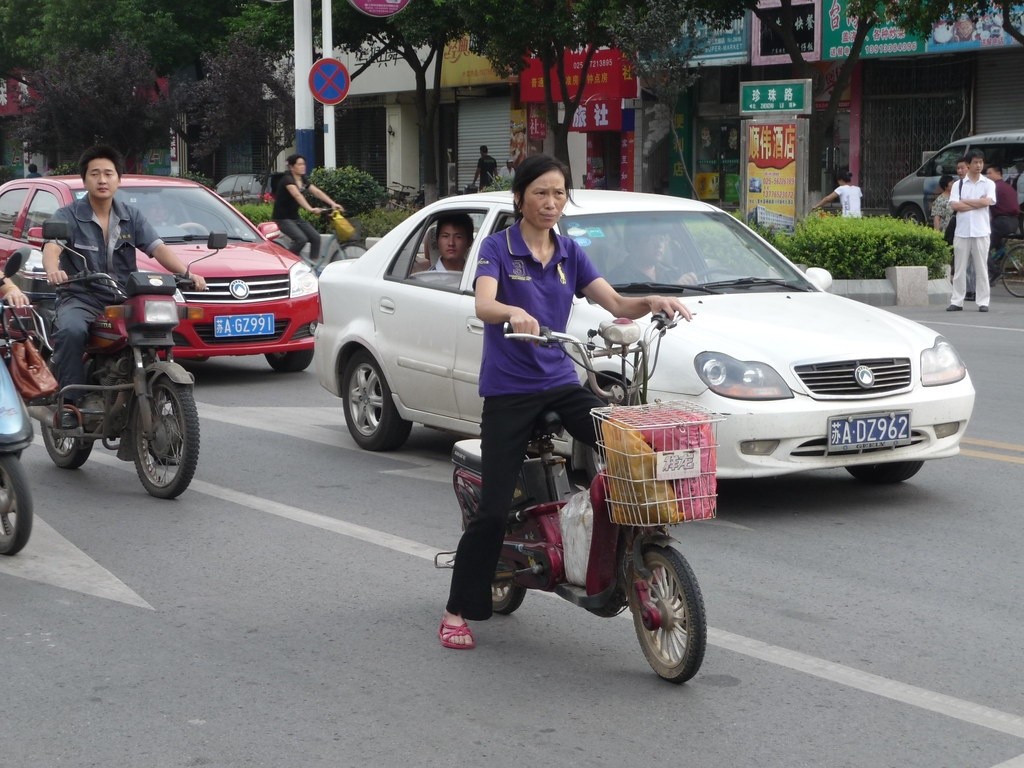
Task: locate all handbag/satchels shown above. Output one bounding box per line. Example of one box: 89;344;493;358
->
2;301;57;398
465;185;478;193
332;210;355;240
944;217;956;244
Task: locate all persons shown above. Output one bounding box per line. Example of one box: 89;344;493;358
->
26;160;60;178
931;175;955;283
41;143;207;464
0;271;30;308
984;164;1019;285
499;160;515;180
812;171;863;220
946;149;997;311
438;154;693;649
272;154;345;266
423;213;474;288
956;158;977;302
473;145;498;190
609;217;699;284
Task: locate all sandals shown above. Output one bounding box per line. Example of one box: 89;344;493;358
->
439;618;474;648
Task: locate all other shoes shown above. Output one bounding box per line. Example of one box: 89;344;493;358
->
980;305;987;312
947;305;962;311
60;413;77;427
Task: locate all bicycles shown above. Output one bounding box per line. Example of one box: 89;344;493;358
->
386;182;424;211
271;203;368;275
951;224;1024;298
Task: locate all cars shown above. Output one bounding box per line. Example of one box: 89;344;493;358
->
210;173;273;206
313;188;975;486
0;173;320;373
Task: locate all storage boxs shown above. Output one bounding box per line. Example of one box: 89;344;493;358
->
4;246;59;310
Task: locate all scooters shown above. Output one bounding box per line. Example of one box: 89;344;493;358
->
435;305;727;686
0;252;37;556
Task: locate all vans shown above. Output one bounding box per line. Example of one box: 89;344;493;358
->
889;129;1024;228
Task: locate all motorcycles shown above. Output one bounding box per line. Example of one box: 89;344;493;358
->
27;220;227;499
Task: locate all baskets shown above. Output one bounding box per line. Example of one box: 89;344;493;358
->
589;399;726;526
333;217;362;245
